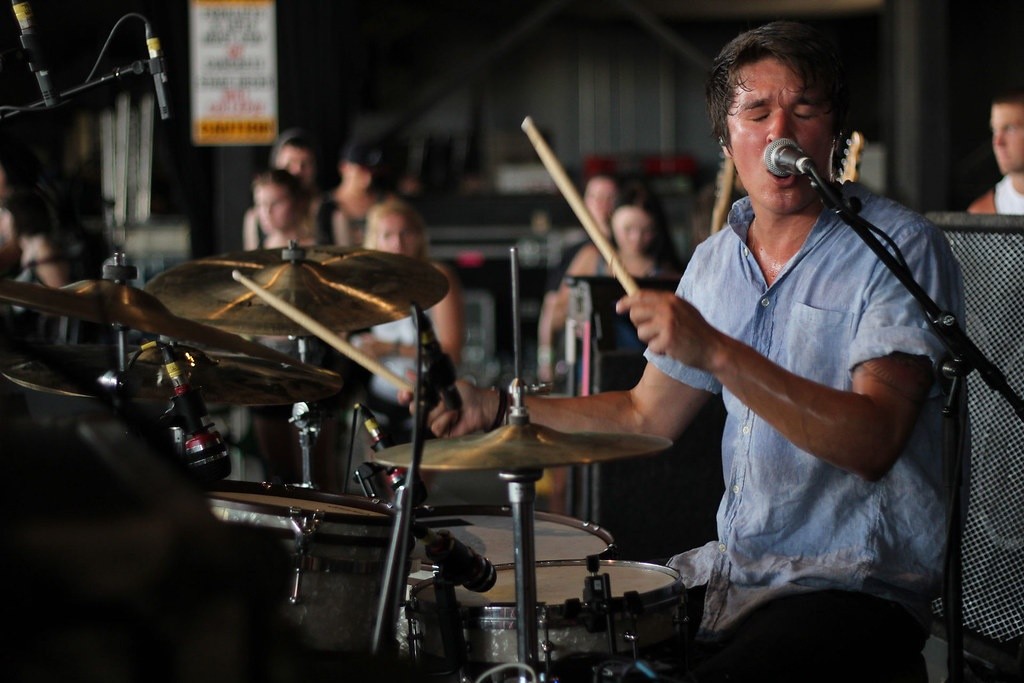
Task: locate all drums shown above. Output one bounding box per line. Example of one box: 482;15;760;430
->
392;502;618;608
205;477;398;655
456;561;690;676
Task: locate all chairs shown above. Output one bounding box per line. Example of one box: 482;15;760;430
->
908;208;1024;683
564;274;728;560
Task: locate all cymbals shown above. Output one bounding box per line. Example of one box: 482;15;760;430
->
136;242;453;339
5;341;338;408
0;269;343;392
366;418;678;474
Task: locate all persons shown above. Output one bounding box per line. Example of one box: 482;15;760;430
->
0;76;1024;507
409;20;969;683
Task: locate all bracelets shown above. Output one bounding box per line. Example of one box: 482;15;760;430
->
484;385;507;432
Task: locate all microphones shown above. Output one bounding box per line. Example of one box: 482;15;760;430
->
157;344;234;487
145;22;177;120
764;138;816;177
408;303;462;422
12;0;61;108
425;529;498;594
360;407;428;509
